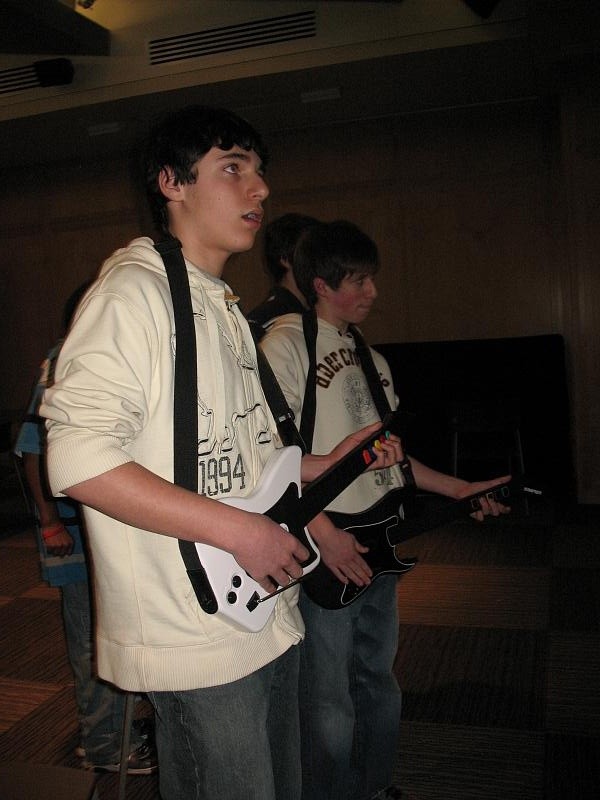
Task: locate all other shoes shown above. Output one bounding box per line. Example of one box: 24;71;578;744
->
376;785;407;800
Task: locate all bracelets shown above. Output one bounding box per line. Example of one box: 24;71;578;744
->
43;524;65;539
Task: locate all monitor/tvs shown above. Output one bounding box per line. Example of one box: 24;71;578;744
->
369;333;578;520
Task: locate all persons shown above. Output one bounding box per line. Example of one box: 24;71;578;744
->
40;101;405;800
14;278;158;775
243;212;510;800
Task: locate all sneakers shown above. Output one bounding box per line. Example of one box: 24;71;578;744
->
76;718;154;756
84;737;159;775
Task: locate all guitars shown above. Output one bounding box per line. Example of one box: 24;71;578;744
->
295;476;548;613
193;421;393;613
